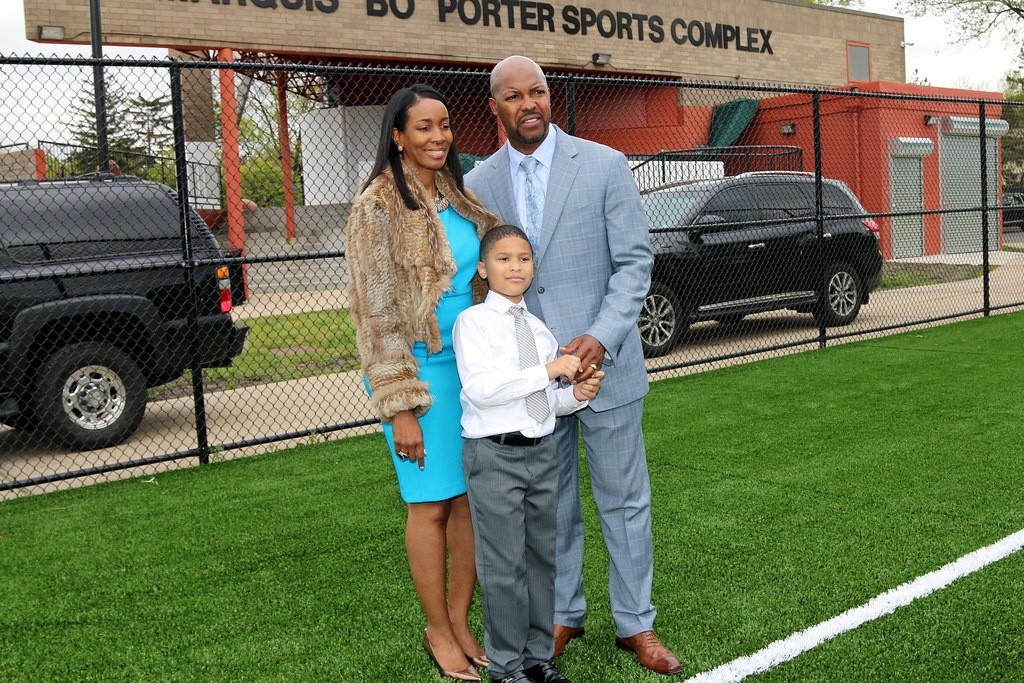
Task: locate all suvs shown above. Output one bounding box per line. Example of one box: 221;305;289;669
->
636;170;883;358
0;172;250;452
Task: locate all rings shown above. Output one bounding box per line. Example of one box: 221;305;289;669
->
590;364;598;370
398;451;409;460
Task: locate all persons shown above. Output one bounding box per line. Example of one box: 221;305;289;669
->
345;83;505;683
465;55;684;676
452;224;605;683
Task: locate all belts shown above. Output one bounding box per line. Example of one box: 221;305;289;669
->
484;433;543;447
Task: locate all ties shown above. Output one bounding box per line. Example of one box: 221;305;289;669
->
520;156;546;265
508;306;550;424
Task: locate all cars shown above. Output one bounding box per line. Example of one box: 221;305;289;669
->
1001;192;1024;231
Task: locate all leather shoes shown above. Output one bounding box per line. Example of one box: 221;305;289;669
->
422;627;490;680
553;624;585;658
491;660;572;683
616;631;682;674
